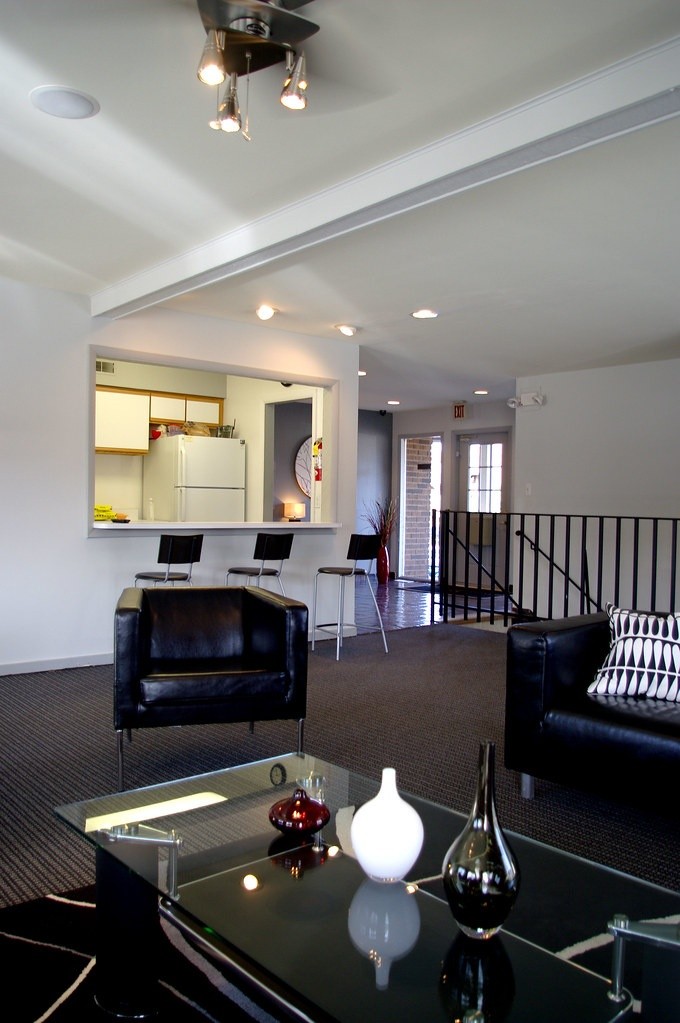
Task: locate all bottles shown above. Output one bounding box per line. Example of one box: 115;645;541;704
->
268;832;328;880
347;879;421;988
436;936;514;1023
147;497;154;522
270;787;331;834
440;742;521;940
350;767;424;884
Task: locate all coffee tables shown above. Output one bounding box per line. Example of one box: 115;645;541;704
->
54;749;680;1023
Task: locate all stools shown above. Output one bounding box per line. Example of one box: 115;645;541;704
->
135;534;204;587
311;534;388;660
224;532;295;597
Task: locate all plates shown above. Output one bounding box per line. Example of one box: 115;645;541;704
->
111;519;130;523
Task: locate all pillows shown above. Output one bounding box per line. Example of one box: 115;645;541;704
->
586;600;680;703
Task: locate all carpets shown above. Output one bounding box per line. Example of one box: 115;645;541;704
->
397;584;509;600
1;814;679;1023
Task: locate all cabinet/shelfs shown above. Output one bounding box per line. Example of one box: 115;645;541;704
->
94;385;225;455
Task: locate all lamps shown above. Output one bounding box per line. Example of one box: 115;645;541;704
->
196;0;320;142
507;388;544;408
284;502;305;522
336;325;357;337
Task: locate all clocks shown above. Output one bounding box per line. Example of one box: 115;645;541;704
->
269;762;285;786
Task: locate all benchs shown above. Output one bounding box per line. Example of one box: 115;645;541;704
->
504;608;680;817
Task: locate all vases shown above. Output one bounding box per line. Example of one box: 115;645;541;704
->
347;876;421;990
268;832;328;880
442;740;521;939
377;540;390;589
268;789;330;835
438;928;516;1023
351;767;425;883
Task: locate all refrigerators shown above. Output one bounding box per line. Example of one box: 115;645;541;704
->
142;436;246;523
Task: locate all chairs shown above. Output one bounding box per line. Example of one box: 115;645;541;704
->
112;586;309;793
